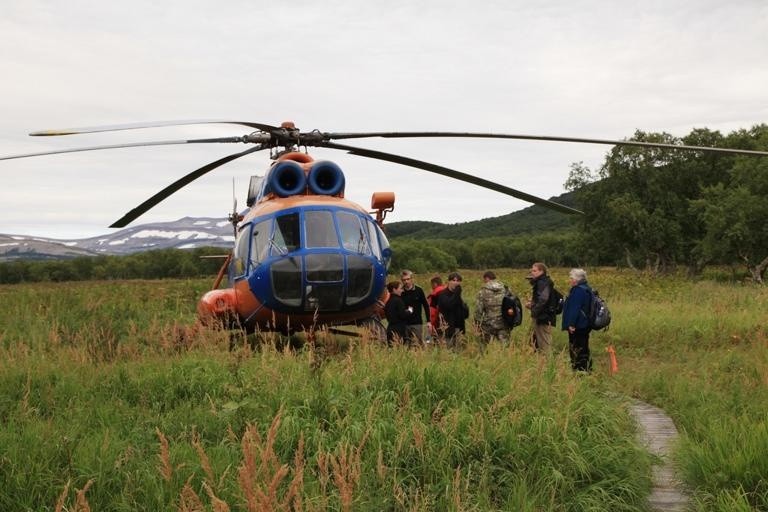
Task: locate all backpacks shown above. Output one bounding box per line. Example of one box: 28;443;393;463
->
501;285;523;331
578;285;612;332
547;288;564;315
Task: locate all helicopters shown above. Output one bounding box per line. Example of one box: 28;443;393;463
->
0;117;768;354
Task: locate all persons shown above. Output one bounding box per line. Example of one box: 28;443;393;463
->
345;229;372;252
383;262;612;373
